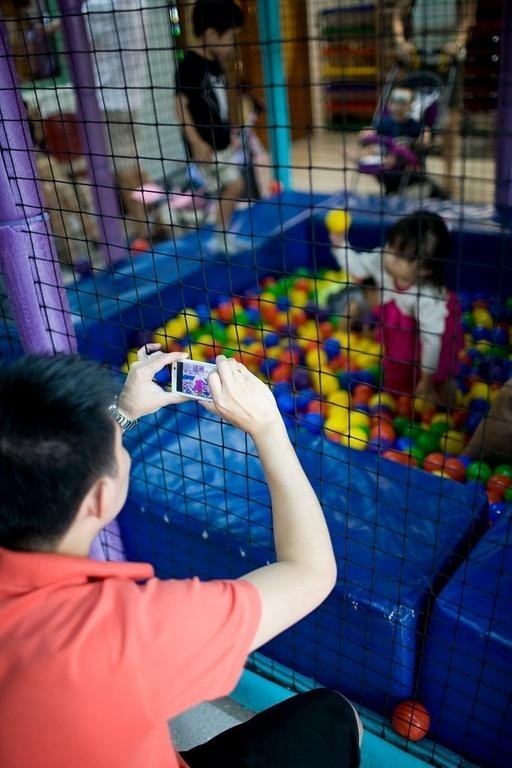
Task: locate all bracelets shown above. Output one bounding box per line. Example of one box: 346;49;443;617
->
107;402;138;432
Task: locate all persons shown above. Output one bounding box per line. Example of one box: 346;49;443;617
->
342;83;420;174
173;1;250;259
0;348;368;767
391;0;478;202
30;0;153;166
328;207;466;408
185;56;272;200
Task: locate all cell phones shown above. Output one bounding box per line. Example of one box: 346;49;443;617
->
171;359;220;404
